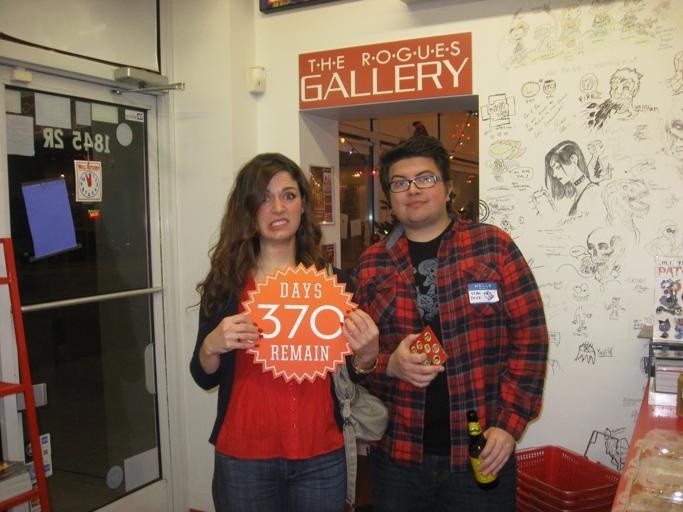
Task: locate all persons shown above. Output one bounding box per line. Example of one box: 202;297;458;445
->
544;139;602;218
543;80;557;99
608;67;643;119
586;227;619;273
347;133;550;511
188;151;380;512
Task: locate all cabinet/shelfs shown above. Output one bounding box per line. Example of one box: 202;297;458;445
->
0;237;50;512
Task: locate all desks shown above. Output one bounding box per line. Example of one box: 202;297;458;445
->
606;377;683;512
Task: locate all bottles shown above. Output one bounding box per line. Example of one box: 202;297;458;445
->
463;406;501;489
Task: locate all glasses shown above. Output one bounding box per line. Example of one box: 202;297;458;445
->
385;173;446;194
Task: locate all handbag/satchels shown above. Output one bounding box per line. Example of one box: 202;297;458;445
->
326;261;392;508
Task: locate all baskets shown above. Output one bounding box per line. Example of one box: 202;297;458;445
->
514;443;622;512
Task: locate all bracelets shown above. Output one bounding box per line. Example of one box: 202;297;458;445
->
351;352;378;374
